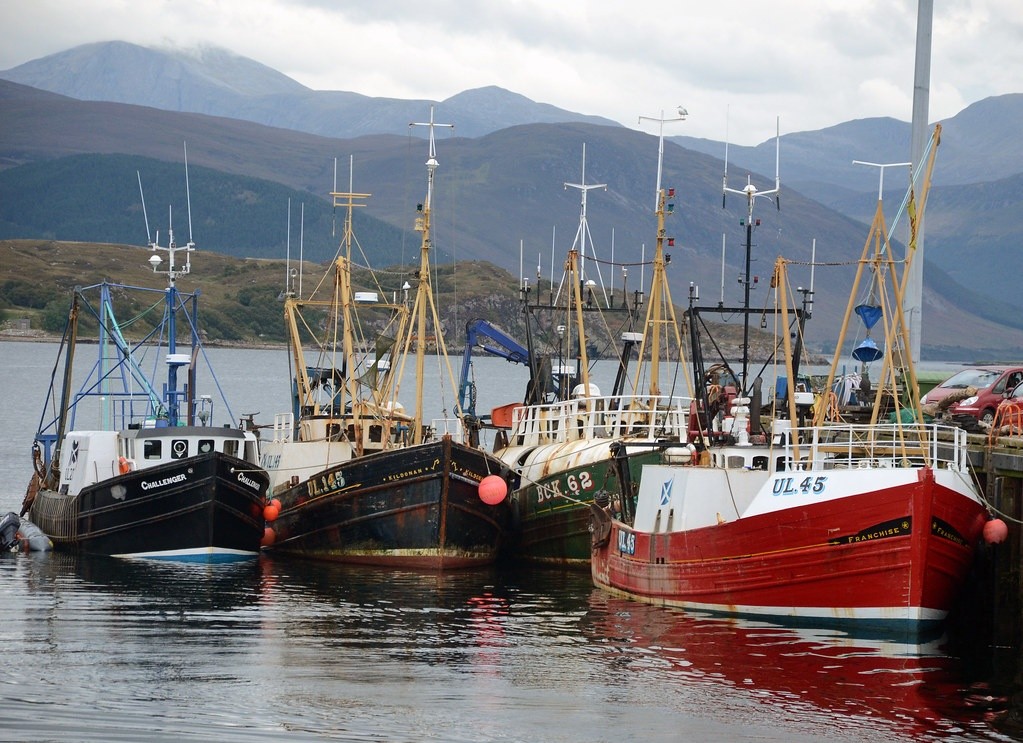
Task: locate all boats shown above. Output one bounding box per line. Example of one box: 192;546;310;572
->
260;101;521;570
492;106;782;566
589;123;993;658
19;139;271;565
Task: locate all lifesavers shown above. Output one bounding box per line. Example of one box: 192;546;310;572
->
118;456;128;475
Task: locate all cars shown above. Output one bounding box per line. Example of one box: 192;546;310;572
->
920;365;1023;434
997;379;1023;435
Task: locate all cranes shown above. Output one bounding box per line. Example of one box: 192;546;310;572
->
451;317;577;456
